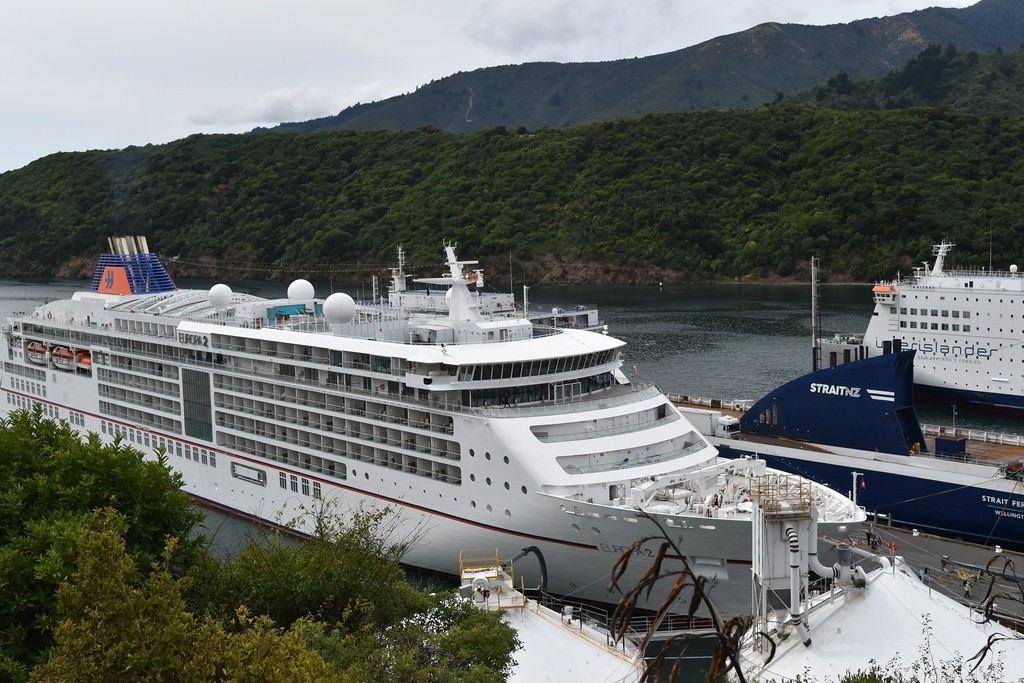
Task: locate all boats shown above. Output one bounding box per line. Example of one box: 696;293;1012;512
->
25;341;48;366
51;346;76;371
75;351;93;375
357;548;650;683
720;471;1024;683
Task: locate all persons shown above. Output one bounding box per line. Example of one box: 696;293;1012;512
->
866;530;872;546
963;580;972;598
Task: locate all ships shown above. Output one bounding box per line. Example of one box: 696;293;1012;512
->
0;233;870;621
660;240;1024;636
